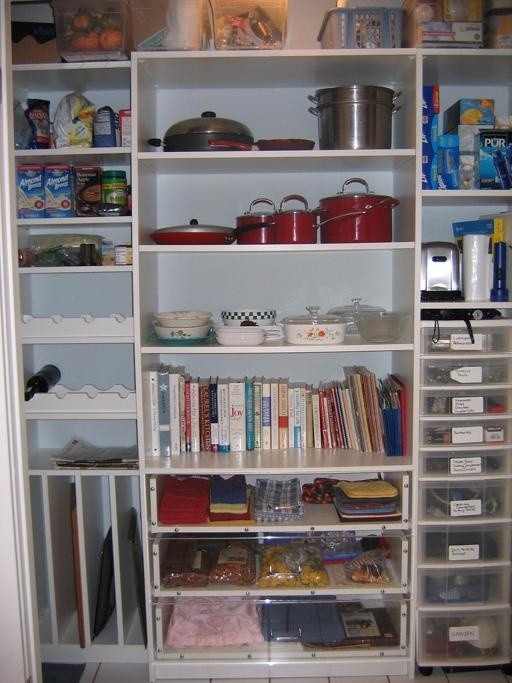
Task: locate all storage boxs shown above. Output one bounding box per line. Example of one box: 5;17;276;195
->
484;8;512;49
51;0;133;61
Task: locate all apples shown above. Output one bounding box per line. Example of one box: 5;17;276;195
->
65;12;122;52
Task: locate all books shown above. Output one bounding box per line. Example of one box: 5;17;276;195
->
148;360;408;457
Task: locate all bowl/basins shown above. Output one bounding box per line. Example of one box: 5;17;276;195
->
151;298;410;345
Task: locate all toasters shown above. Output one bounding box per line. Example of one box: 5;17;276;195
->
421;241;465;302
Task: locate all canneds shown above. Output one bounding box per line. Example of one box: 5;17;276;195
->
100;169;127;207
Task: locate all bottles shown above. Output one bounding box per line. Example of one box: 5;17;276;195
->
25;365;61;402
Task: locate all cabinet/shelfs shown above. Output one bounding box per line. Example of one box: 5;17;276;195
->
418;358;510;387
417;389;509;417
417;566;511;608
417;419;511;447
415;326;512;672
417;326;510;357
418;449;509;478
1;47;512;683
416;524;512;566
417;608;510;666
416;479;511;521
418;50;511;193
416;307;510;325
417;196;510;306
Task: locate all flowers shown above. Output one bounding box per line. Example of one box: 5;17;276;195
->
168;330;190;339
297;326;340;344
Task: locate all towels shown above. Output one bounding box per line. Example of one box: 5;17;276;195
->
156;474;304;528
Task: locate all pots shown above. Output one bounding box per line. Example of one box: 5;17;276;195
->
148;177;400;244
147;84;403;152
